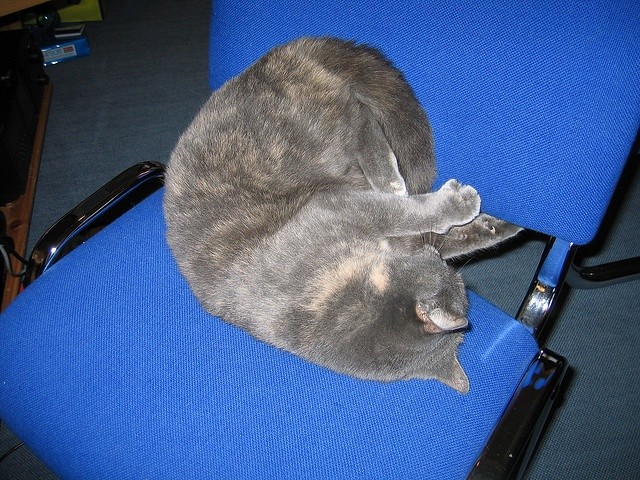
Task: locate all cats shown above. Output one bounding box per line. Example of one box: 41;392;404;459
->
160;34;527;396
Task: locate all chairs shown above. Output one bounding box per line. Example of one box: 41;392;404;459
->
0;2;639;480
